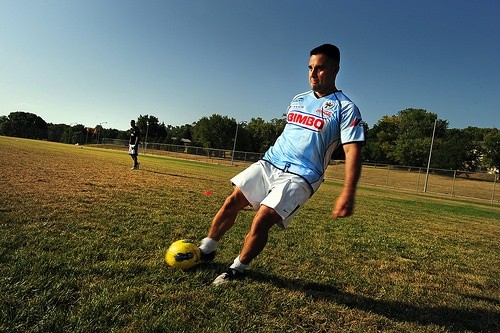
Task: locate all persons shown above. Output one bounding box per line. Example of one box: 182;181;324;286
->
128;120;140;170
199;43;365;287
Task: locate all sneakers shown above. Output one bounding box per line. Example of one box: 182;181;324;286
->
199;248;216;261
211;268;245;290
136;162;140;168
130;166;136;170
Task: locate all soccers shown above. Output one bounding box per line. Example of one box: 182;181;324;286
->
164;240;201;270
